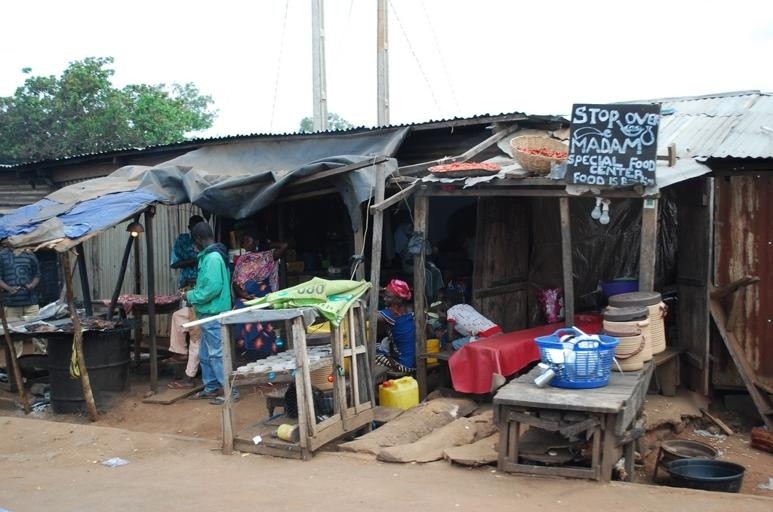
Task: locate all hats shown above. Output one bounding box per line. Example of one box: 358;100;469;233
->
387;279;412;300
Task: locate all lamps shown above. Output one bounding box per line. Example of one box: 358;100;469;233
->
588;196;613;225
125;213;143;238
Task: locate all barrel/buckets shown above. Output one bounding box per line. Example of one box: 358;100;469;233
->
599;306;654;370
45;328;132;414
380;377;421;410
608;291;667;357
426;338;442;363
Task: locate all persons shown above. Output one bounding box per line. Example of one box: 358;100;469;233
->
0;248;47;360
177;222;241;406
392;214;445;304
437;291;505;352
167;215;207;308
425;286;452;339
363;277;418;389
159;306;202;389
227;227;290;364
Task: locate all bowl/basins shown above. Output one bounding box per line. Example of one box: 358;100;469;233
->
600;280;638;297
236;344;333;372
426;162;503;178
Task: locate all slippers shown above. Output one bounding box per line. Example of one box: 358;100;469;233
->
160;356;187;365
210;395;239;405
167;377;194;388
190;392;217;400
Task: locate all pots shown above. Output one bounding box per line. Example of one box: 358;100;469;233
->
660;438;747;493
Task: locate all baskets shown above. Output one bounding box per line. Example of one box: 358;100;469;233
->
533;327;619;390
510;134;570;175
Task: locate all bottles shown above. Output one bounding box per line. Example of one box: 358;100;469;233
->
255;239;268;252
448;318;455;343
533;366;559;388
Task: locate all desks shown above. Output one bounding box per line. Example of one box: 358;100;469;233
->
89;293;182;384
